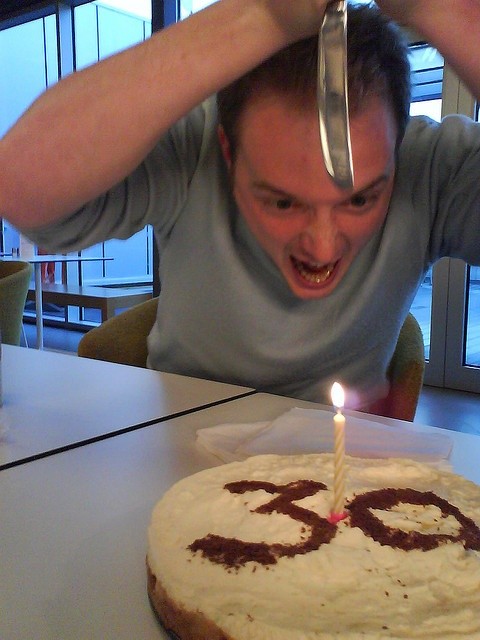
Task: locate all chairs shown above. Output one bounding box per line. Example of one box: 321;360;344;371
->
78;294;424;421
0;261;32;348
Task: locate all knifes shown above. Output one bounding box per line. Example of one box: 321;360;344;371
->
315;0;356;191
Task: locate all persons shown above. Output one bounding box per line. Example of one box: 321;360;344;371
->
0;0;480;417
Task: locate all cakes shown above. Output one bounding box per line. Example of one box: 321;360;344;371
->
146;452;480;640
